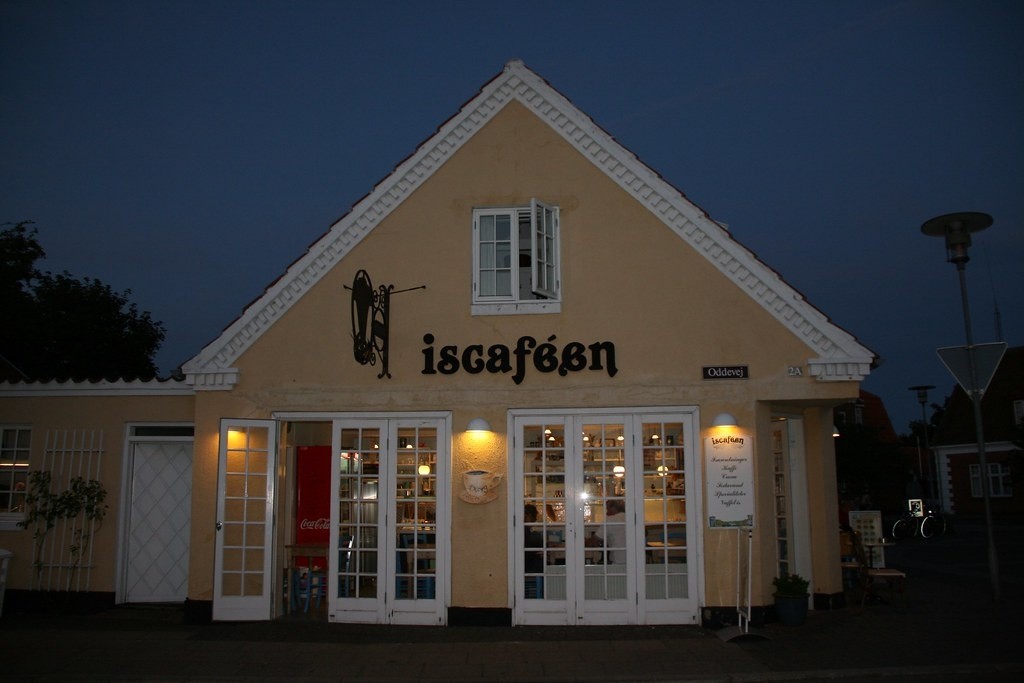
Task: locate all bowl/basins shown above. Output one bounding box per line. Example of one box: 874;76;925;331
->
463;470;501;497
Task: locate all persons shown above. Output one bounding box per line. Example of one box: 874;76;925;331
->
839;489;873;576
12;482;26;512
583;500;627;564
524;505;552;574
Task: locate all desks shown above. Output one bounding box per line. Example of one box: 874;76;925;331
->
284;545;328;616
400;523;436;535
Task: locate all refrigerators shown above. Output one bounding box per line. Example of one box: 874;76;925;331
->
295;445;364;575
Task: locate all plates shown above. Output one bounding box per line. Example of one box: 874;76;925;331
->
459;490;498;504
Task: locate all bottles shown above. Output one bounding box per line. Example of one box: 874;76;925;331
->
882;537;885;544
411;478;428;496
400;438;406;448
535;451;564;484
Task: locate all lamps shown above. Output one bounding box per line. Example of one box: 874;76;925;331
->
419;452;430;476
613;446;625;478
466;418;493;432
712;412;738;429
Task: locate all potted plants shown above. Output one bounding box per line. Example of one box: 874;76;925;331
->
773;572;810;626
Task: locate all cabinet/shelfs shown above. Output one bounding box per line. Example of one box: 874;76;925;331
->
342;434;436;537
524;426;686;564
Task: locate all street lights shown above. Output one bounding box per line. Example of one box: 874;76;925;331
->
920;211;1003;615
907;385;937;479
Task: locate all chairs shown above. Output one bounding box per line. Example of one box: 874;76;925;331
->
395;531;436;599
842;535;906;614
304;535;354;613
525;563;544;600
283;568;301;614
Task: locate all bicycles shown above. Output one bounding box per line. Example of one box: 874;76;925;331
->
891;502;943;541
